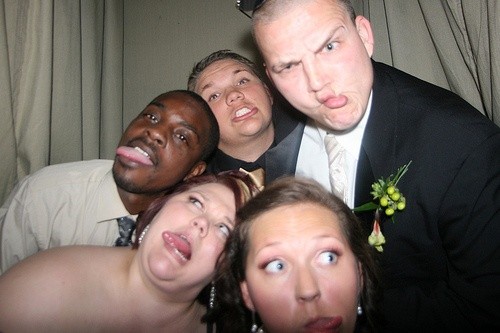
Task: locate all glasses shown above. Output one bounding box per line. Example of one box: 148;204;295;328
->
236;0;263;18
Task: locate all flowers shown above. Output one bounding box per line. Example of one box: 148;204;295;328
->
349;160;413;252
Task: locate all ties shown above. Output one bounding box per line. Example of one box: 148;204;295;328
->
240;167;265;196
114;216;137;246
324;129;346;202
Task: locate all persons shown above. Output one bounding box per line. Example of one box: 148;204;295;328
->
236;1;500;333
0;169;259;333
0;89;220;278
228;175;382;333
187;49;306;192
